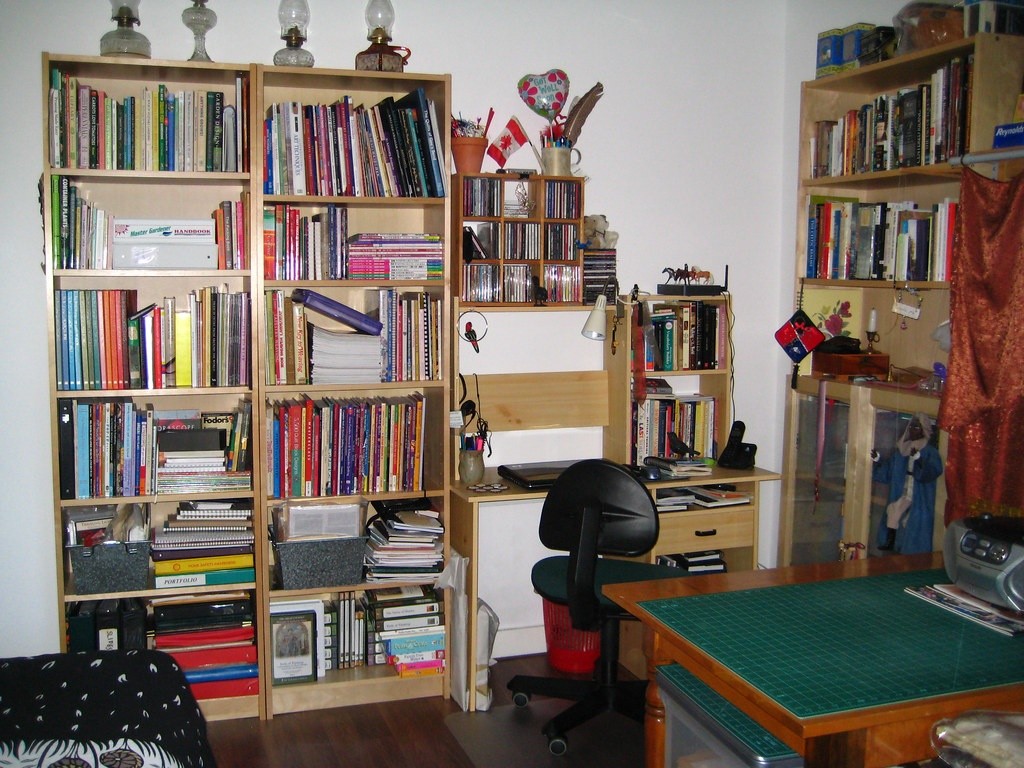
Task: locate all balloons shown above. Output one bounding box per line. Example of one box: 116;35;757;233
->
517;69;570;124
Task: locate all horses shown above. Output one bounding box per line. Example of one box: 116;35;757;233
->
662;263;714;285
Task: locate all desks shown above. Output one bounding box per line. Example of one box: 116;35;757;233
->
602;551;1023;768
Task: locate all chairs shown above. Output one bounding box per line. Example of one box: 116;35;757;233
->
507;459;694;756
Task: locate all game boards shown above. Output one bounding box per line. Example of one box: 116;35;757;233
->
655;661;799;759
635;566;1024;721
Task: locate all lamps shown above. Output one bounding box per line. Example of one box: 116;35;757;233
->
181;0;217;62
356;0;412;72
273;0;314;67
98;0;152;59
581;275;624;340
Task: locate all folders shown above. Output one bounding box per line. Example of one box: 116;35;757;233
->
189;678;260;700
170;646;258;667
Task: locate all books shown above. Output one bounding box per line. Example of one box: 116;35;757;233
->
51;174;251;270
804;194;959;282
263;88;450;197
462;177;616;306
656;550;728;576
809;54;974;180
631;300;726;372
264;287;442;386
992;76;1024;149
903;584;1024;637
263;203;445;280
655;484;754;513
56;391;446;700
54;282;252;391
48;68;250;173
630;377;720;476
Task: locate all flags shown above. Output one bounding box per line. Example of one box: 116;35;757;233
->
487;116;530;168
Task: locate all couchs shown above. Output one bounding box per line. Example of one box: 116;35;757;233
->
0;649;219;768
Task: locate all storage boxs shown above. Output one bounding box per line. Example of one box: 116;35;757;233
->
66;533;153;595
652;667;804;768
113;244;220;271
815;26;843;78
267;524;370;591
841;23;877;71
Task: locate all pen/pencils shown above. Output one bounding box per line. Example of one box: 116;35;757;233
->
460;434;483;451
540;131;572;149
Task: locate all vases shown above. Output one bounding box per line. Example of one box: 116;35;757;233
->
451;137;489;173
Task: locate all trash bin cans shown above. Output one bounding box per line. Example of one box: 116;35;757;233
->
543;597;600;674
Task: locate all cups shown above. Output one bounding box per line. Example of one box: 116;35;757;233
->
458;448;485;484
451;137;488;174
542;147;581;176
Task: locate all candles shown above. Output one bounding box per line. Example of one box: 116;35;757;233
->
867;306;878;331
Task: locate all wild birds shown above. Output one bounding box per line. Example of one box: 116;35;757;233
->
666;432;702;460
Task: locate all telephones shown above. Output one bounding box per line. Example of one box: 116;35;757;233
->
717;421;757;469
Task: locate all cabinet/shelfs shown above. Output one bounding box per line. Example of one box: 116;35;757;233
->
39;56;781;720
788;34;1024;575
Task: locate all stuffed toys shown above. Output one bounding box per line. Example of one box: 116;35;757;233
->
584;215;618;249
